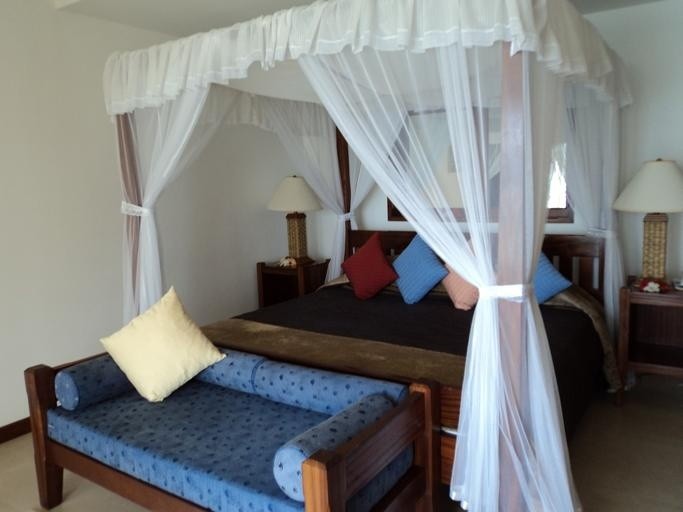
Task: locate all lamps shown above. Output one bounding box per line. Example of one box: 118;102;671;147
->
266;175;322;264
612;158;683;289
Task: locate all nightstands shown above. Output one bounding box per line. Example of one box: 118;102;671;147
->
255;261;330;307
614;274;683;385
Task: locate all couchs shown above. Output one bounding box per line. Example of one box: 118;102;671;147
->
23;350;433;512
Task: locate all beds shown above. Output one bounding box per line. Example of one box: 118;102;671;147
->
100;0;632;512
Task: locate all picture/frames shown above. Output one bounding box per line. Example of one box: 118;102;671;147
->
387;101;578;222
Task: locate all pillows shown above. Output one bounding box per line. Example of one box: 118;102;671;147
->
53;354;131;410
195;346;266;396
504;244;573;306
390;233;449;305
100;286;226;401
441;237;489;311
272;395;395;504
340;232;399;300
252;359;408;417
341;267;579;308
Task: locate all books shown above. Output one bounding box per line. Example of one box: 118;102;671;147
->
673;279;683;291
